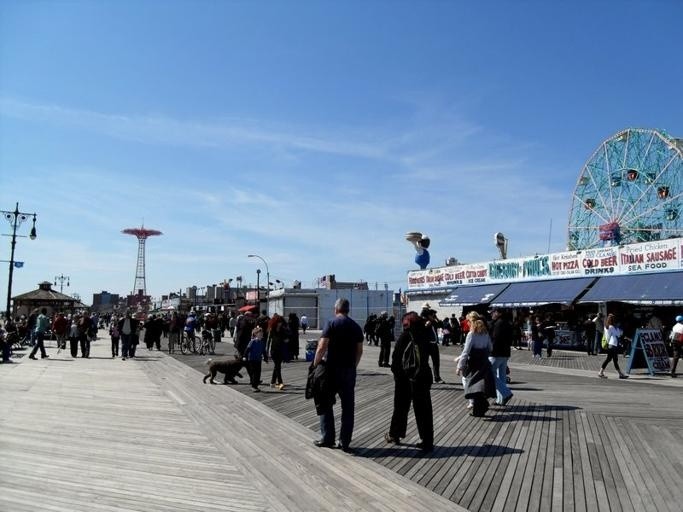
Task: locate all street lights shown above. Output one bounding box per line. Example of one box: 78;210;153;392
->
276;279;284;289
270;282;275;289
200;286;205;296
247;254;269;315
53;273;69;295
0;203;37;315
219;283;224;288
255;269;261;299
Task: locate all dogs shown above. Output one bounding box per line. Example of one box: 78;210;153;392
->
202;355;244;384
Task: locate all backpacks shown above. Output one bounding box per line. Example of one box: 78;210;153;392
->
391;332;420;380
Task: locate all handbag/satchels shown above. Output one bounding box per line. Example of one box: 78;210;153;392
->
602;335;608;349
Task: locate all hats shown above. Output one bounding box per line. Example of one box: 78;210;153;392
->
487;306;500;313
675;315;683;321
676;316;682;321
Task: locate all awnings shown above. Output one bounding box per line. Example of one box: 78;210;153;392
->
238;305;257;311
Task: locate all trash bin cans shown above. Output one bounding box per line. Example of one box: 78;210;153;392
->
305;340;318;362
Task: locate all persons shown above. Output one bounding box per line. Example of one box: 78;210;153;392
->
310;297;364;452
512;308;555;359
440;313;470;347
575;312;604;355
668;315;682;378
365;311;395;367
144;308;237;355
597;314;632;379
489;309;513;406
453;311;494;418
236;310;309;392
646;313;661;329
382;310;436;454
1;307;144;364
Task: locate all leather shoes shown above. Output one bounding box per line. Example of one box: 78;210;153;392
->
314;440;333;448
385;431;400;444
339;439;348;450
416;440;434;449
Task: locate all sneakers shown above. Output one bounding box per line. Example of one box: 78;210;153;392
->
467;393;513;417
252;387;260;392
438;380;445;384
599;374;607;378
270;383;276;387
619;375;629;378
279;383;284;390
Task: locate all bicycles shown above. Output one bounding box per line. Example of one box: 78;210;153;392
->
202;326;216;355
180;327;201;354
